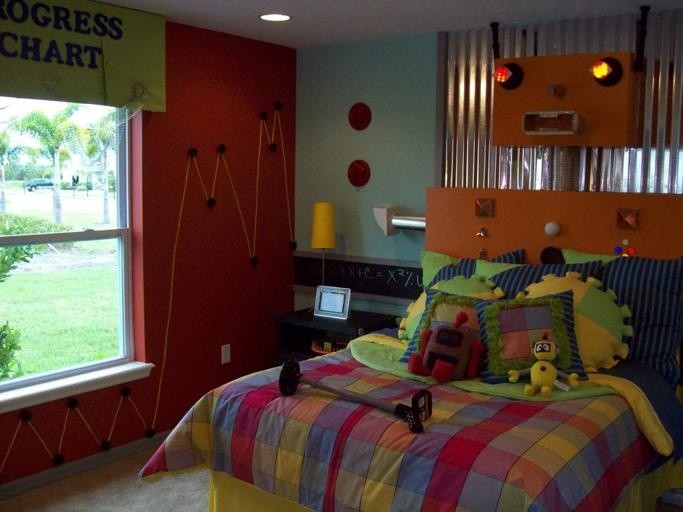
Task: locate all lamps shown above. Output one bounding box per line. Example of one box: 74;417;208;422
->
306;200;336;313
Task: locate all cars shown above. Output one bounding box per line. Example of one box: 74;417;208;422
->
22;178;54;192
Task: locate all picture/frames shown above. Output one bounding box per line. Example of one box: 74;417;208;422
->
311;284;353;321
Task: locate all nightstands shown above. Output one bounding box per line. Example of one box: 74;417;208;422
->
270;305;400;366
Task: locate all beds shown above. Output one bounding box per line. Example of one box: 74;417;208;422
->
137;183;682;510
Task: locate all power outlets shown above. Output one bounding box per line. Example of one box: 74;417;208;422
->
220;343;230;366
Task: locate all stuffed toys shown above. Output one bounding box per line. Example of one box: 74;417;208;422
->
408;310;482;384
508;332;579;399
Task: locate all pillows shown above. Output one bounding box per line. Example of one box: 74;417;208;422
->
395;246;681;384
